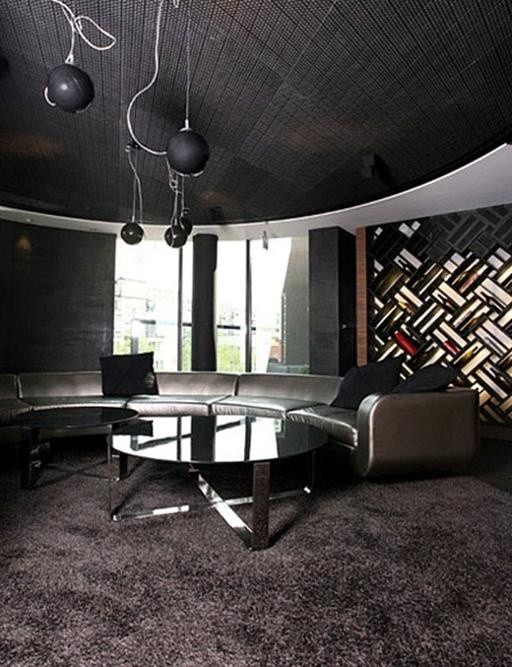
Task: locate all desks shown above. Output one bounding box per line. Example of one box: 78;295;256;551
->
108;416;328;551
1;404;143;488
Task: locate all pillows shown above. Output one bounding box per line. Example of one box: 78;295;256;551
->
98;349;159;396
392;361;462;391
328;355;405;409
